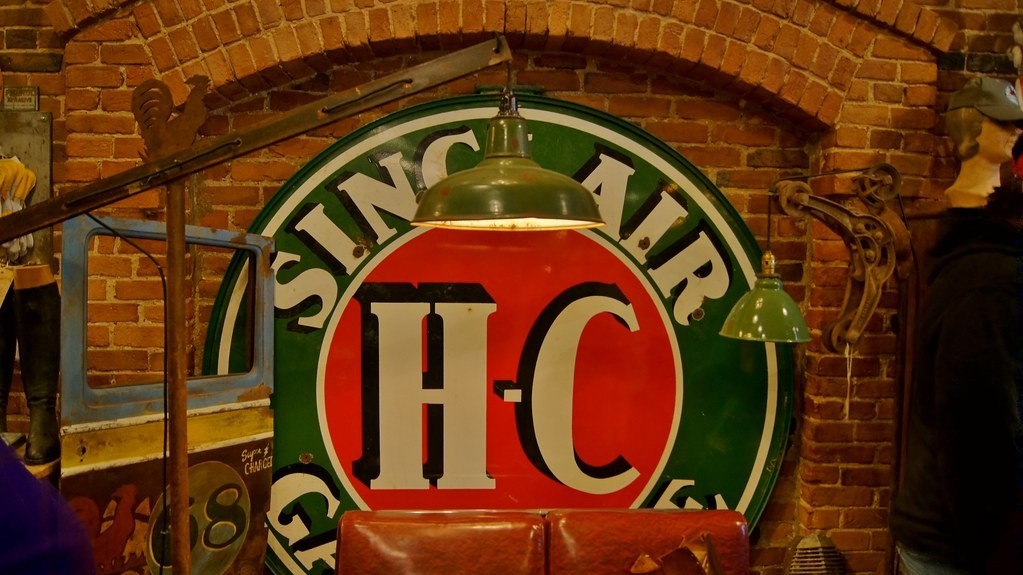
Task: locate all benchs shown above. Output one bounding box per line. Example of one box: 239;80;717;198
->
335;508;751;575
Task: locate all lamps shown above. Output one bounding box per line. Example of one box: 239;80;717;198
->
0;33;605;245
717;161;913;354
